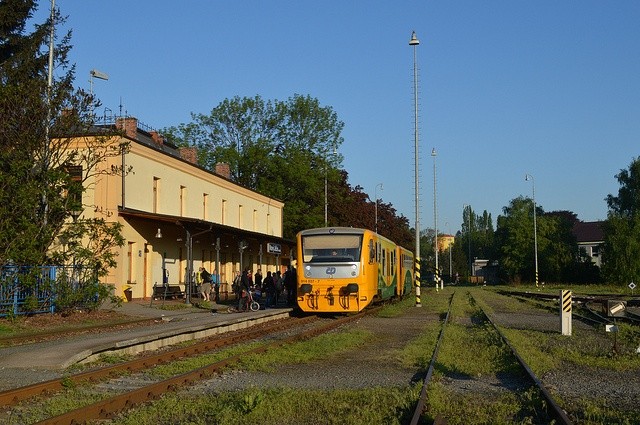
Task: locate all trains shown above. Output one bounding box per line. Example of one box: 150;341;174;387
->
296;227;440;318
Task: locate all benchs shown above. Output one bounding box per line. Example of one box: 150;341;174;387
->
153;286;184;300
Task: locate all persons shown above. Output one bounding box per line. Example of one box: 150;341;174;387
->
292;268;297;305
249;271;254;288
234;271;242;299
263;271;274;308
331;251;338;256
255;269;262;296
272;273;278;307
236;267;251;312
276;271;284;306
282;265;292;306
196;267;203;287
200;268;212;302
210;270;220;301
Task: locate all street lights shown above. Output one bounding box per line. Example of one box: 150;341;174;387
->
323;140;338;227
526;174;540;289
374;182;384;234
410;31;422;307
462;202;473;284
431;146;438;295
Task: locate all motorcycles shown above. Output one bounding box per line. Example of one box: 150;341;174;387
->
227;285;260;314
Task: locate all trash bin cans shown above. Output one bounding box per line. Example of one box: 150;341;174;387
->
121;285;133;302
